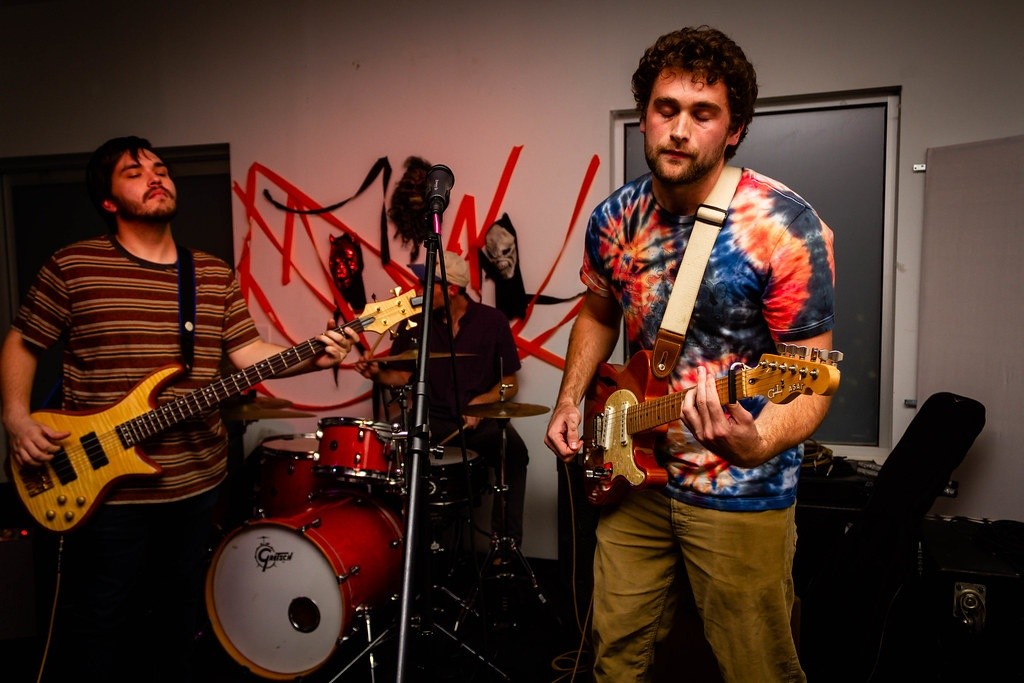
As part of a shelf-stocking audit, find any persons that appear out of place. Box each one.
[0,136,361,683]
[354,252,529,557]
[543,25,836,683]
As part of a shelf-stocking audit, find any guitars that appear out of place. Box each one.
[580,343,845,510]
[6,287,424,535]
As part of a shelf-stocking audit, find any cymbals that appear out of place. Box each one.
[226,395,293,409]
[461,399,552,417]
[225,404,317,420]
[358,346,478,363]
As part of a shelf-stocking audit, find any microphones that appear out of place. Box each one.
[424,164,454,213]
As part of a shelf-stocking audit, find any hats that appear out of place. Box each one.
[406,251,469,288]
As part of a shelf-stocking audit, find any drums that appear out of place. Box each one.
[423,447,481,508]
[254,432,320,510]
[314,416,402,486]
[201,502,408,678]
